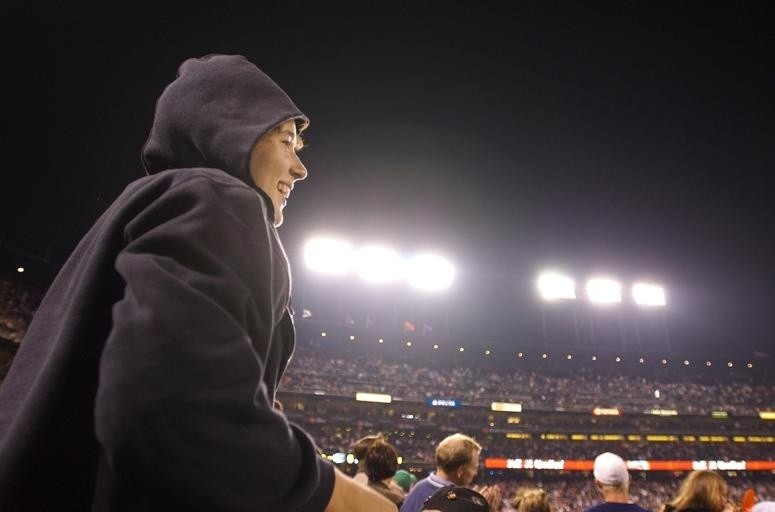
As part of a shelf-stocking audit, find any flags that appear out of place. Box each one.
[403,322,416,332]
[424,324,433,331]
[302,309,311,318]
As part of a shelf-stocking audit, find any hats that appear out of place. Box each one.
[593,451,629,485]
[423,486,489,512]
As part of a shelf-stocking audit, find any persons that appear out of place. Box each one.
[276,351,775,511]
[0,283,51,386]
[1,51,399,512]
